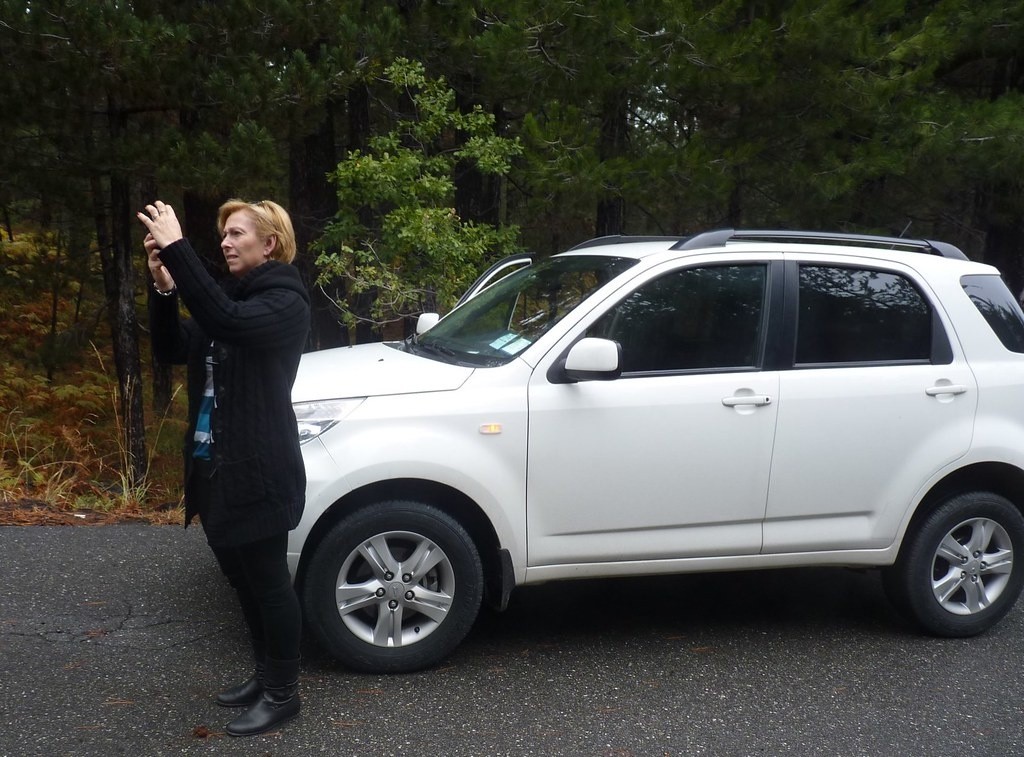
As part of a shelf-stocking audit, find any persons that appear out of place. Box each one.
[137,199,311,736]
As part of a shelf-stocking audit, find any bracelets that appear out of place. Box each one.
[152,280,177,295]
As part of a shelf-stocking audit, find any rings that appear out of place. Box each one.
[151,213,159,220]
[159,210,166,213]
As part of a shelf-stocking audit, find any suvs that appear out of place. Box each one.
[287,226,1024,678]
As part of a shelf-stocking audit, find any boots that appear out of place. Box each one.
[215,670,301,737]
